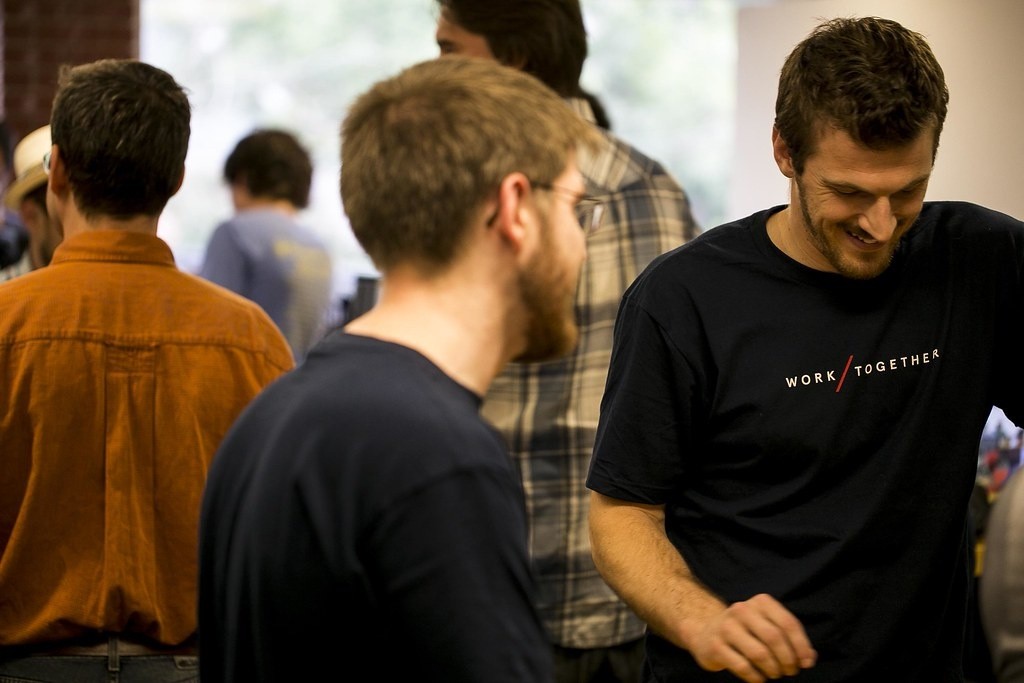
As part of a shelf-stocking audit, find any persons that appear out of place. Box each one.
[195,54,606,682]
[0,56,298,683]
[436,0,707,683]
[0,120,64,283]
[194,127,344,369]
[584,13,1023,683]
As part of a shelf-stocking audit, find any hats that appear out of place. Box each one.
[2,124,52,212]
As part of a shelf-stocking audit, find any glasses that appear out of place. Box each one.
[486,179,606,239]
[43,151,51,173]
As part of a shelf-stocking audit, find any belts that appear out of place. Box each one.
[0,627,199,656]
